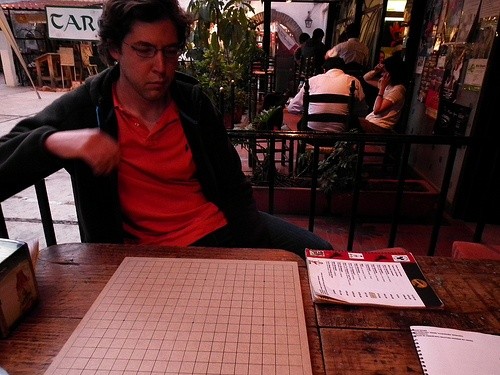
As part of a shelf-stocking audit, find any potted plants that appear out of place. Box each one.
[231,106,440,216]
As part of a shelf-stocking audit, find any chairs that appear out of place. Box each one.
[248,79,410,176]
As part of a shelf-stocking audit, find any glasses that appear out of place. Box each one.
[122,40,182,61]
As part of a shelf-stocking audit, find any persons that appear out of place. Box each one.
[325,26,370,72]
[0,0,332,254]
[288,56,367,147]
[452,59,463,89]
[304,28,325,57]
[359,56,406,134]
[294,33,310,60]
[380,51,385,61]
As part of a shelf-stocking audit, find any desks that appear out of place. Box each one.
[305,249,500,375]
[1,244,325,375]
[33,53,74,90]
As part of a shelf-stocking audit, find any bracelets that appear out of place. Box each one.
[377,94,383,97]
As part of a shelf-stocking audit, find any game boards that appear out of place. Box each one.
[42,257,313,374]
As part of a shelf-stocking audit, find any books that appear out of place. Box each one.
[305,248,444,310]
